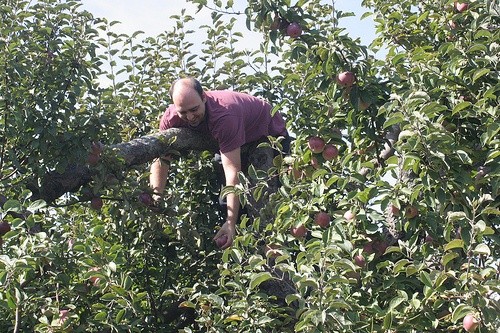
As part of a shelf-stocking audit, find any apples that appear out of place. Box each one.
[0,1,490,333]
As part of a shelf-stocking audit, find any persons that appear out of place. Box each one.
[151,78,291,249]
